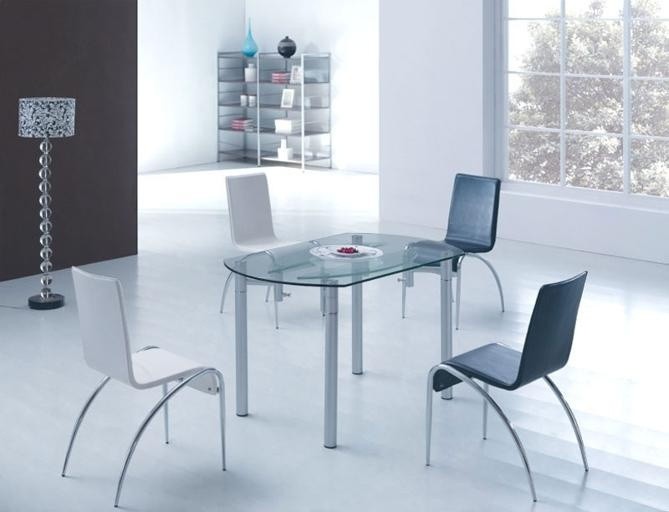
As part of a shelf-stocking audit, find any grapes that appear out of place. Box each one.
[342,247,354,252]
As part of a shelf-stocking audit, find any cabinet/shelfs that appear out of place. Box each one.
[216,51,333,170]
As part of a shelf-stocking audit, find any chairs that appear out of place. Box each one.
[60,265,227,507]
[399,173,505,331]
[424,269,590,503]
[219,172,325,331]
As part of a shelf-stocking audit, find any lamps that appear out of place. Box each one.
[17,97,76,312]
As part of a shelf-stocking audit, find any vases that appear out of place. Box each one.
[278,36,295,58]
[242,18,256,57]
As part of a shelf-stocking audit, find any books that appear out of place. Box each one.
[231,118,254,131]
[271,72,290,84]
[274,118,306,134]
